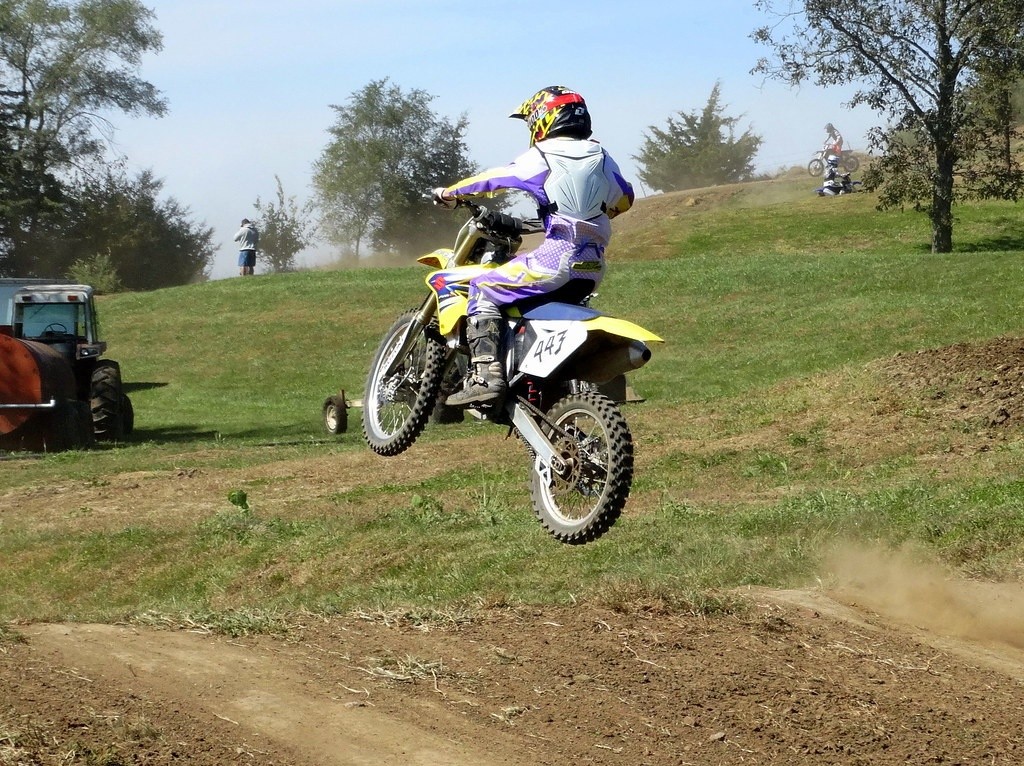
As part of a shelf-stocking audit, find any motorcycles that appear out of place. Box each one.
[808,144,859,177]
[814,172,864,197]
[361,193,666,545]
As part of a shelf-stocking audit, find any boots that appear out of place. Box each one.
[445,316,504,406]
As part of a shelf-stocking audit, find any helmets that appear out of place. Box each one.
[828,155,839,168]
[508,84,593,148]
[824,123,833,133]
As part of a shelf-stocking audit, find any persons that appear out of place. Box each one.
[823,155,849,196]
[234,218,259,275]
[431,85,634,405]
[823,123,843,157]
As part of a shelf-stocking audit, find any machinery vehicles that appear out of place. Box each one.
[0,285,123,443]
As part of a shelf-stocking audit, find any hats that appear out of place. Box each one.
[241,219,250,227]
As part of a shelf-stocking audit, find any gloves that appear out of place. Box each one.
[422,188,457,211]
